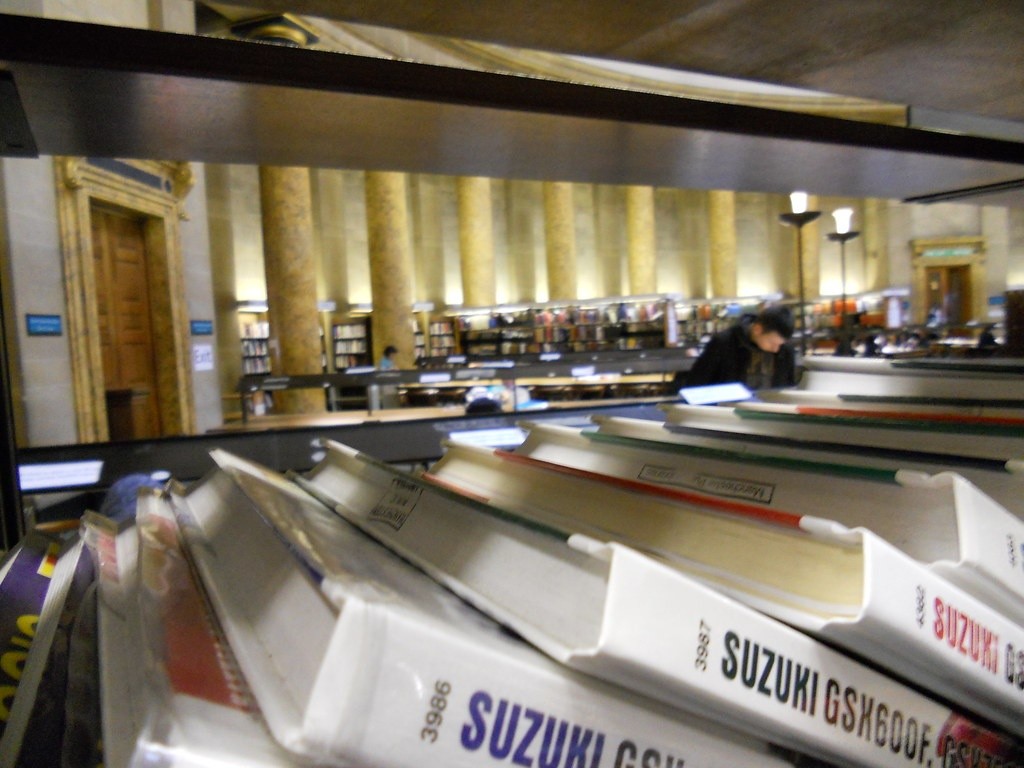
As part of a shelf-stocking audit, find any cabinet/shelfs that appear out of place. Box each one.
[0,0,1024,563]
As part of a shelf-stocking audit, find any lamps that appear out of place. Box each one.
[826,208,860,356]
[777,191,822,356]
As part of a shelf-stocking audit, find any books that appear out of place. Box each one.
[1,357,1024,768]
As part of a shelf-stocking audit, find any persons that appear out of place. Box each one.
[616,303,628,349]
[378,346,403,408]
[686,305,795,389]
